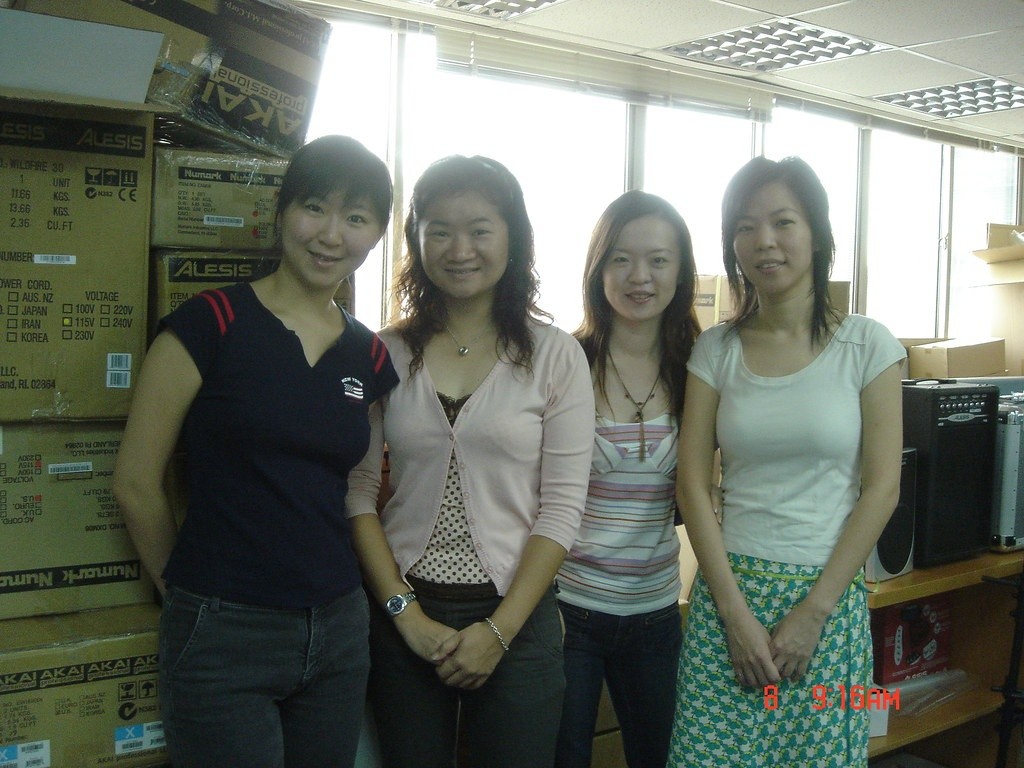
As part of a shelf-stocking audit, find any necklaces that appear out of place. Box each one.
[439,313,492,357]
[605,343,666,463]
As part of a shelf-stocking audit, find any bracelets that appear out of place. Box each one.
[486,617,509,652]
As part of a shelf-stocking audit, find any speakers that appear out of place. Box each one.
[861,383,1001,582]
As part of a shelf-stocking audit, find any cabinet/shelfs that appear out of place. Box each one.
[591,521,1024,768]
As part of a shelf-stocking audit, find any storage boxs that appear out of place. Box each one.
[909,336,1009,379]
[1,0,332,767]
[694,274,852,332]
[972,222,1024,376]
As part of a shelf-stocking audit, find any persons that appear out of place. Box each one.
[109,137,399,768]
[554,188,721,768]
[661,153,908,768]
[343,153,596,768]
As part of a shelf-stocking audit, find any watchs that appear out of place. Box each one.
[382,590,417,619]
